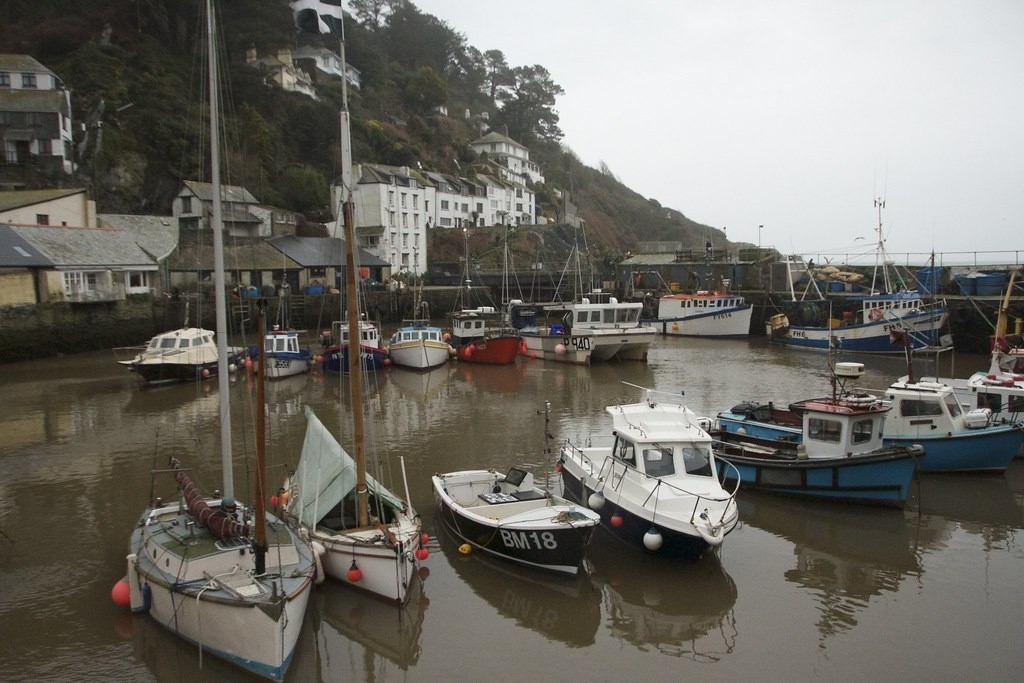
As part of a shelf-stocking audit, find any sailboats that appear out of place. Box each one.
[110,0,429,683]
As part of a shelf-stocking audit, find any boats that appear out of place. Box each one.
[765,162,954,353]
[555,380,741,556]
[638,224,753,339]
[111,163,657,384]
[431,399,601,581]
[696,253,1024,510]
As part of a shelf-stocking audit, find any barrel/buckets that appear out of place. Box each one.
[670,282,681,291]
[915,266,1006,296]
[827,319,841,328]
[551,324,563,335]
[990,335,1006,354]
[539,327,551,335]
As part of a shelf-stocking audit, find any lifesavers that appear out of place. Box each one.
[1002,371,1024,381]
[272,331,287,334]
[1008,348,1024,354]
[847,395,877,408]
[870,309,882,321]
[984,374,1014,385]
